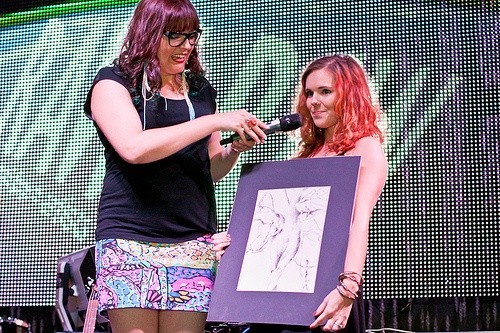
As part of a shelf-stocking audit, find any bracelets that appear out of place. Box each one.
[337,271,365,302]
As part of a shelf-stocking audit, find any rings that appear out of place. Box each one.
[247,116,258,130]
[334,323,343,329]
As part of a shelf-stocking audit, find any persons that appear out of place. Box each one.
[82,1,269,333]
[211,54,390,333]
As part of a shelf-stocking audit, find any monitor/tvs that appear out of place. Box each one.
[56,245,96,313]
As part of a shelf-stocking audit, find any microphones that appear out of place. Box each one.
[220,112,304,145]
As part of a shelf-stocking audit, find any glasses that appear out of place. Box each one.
[162,28,203,47]
